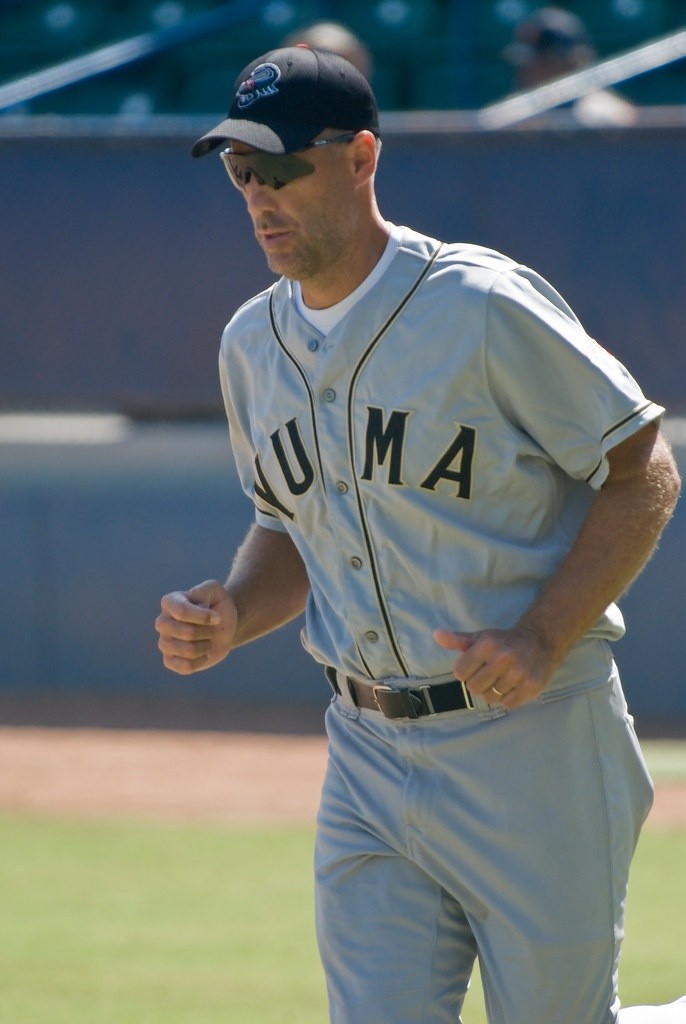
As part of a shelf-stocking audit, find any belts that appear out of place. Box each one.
[333,676,473,719]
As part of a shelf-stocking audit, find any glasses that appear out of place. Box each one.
[220,135,354,190]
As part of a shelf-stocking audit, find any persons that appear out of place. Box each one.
[285,8,636,124]
[155,45,680,1024]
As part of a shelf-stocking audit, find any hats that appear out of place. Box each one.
[190,43,382,158]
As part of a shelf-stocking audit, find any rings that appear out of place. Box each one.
[493,685,503,696]
